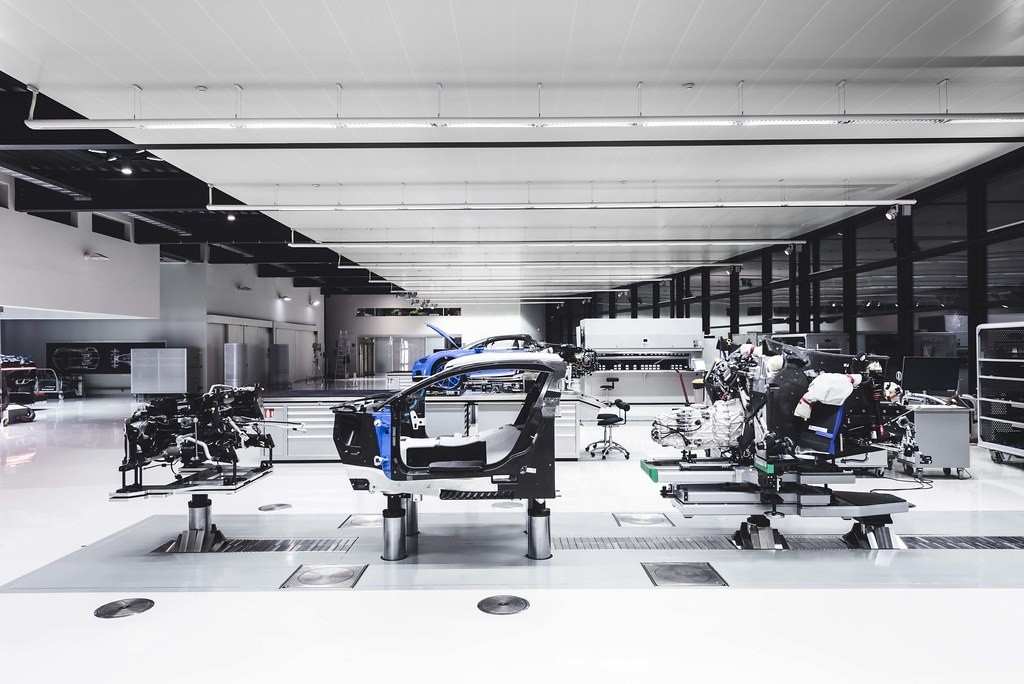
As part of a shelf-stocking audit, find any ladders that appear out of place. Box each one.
[334,329,348,379]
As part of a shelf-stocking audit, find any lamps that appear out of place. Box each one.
[23,78,1024,310]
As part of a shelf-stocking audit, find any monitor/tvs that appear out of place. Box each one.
[901,357,960,391]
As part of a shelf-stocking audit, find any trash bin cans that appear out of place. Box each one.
[691,378,705,404]
